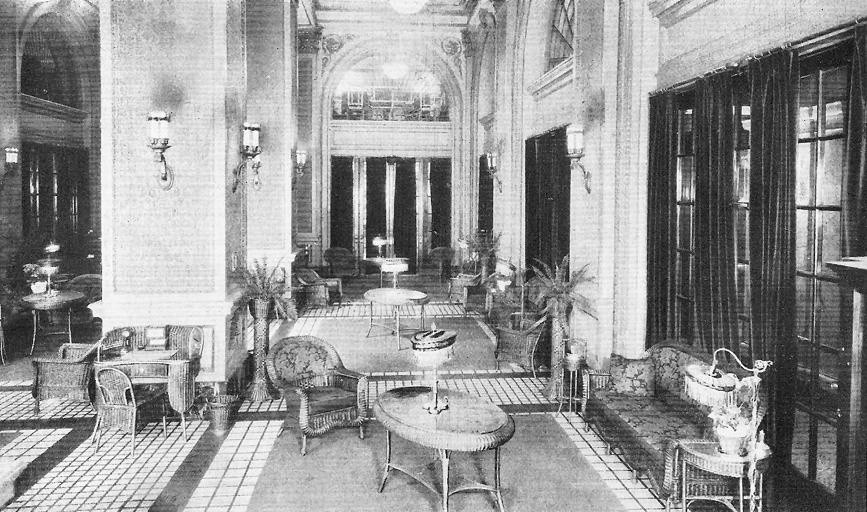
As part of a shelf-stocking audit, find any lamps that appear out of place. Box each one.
[684,346,769,453]
[293,145,307,175]
[232,118,268,190]
[143,108,178,190]
[1,145,23,184]
[564,119,593,191]
[484,145,502,194]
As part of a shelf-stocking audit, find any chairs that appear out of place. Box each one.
[227,228,557,378]
[263,331,368,453]
[0,236,216,463]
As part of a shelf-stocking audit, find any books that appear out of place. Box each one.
[147,339,168,345]
[145,325,168,337]
[144,345,167,351]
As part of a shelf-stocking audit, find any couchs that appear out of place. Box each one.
[577,339,764,512]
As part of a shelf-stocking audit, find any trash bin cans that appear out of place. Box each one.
[207,395,232,436]
[589,368,611,389]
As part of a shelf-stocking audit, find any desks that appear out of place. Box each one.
[663,436,769,512]
[370,384,516,512]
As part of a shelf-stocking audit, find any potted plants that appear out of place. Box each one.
[526,253,599,338]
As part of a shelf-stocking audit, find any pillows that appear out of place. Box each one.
[606,351,656,398]
